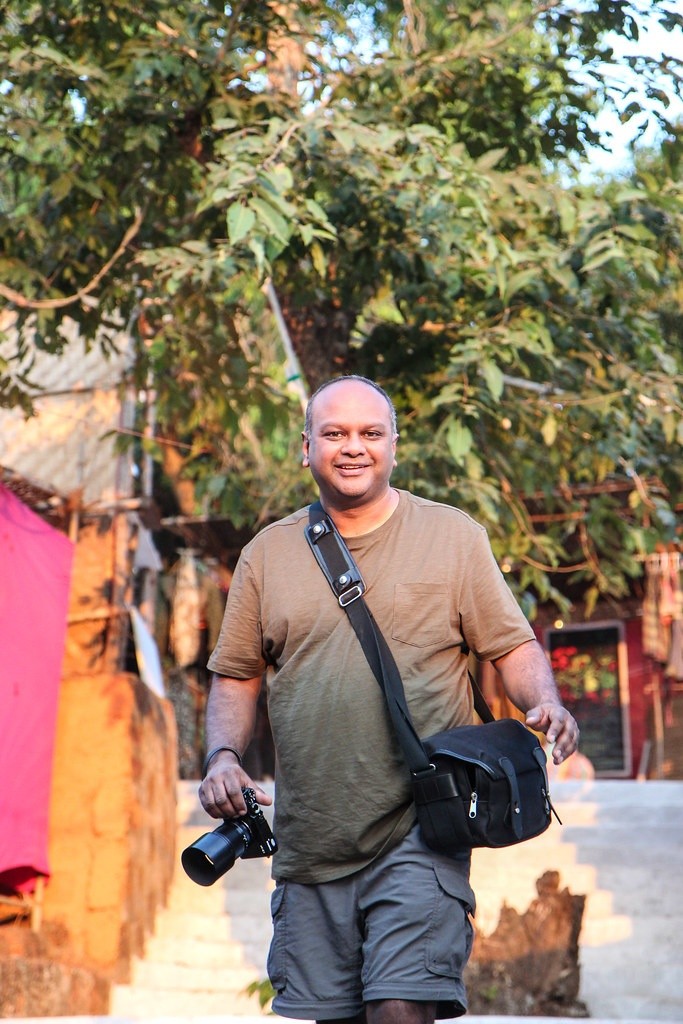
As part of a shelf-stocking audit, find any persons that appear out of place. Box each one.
[199,375,580,1024]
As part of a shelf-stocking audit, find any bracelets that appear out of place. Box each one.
[202,745,243,779]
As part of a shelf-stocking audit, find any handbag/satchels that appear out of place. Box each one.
[410,718,549,853]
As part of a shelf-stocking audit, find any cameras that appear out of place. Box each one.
[181,787,278,887]
